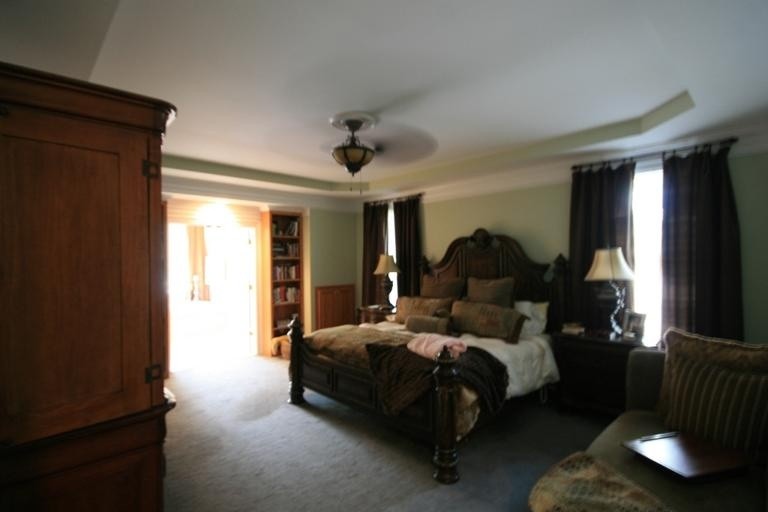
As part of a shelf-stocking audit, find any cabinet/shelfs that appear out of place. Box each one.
[0,58,178,512]
[256,208,304,358]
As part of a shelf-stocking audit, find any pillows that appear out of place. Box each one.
[467,276,515,308]
[395,296,453,324]
[663,352,768,451]
[514,295,548,337]
[658,326,768,451]
[448,299,527,345]
[420,274,465,300]
[405,314,453,336]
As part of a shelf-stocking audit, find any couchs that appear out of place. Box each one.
[584,345,768,512]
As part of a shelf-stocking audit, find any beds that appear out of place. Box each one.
[287,228,573,484]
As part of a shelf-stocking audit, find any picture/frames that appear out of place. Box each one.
[622,311,643,332]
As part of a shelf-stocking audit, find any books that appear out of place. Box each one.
[273,263,300,278]
[273,240,300,257]
[273,219,298,236]
[273,285,299,303]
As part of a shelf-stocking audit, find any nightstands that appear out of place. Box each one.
[355,305,392,326]
[552,312,646,415]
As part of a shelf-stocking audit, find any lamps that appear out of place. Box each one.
[583,246,637,338]
[329,111,375,178]
[372,254,402,310]
